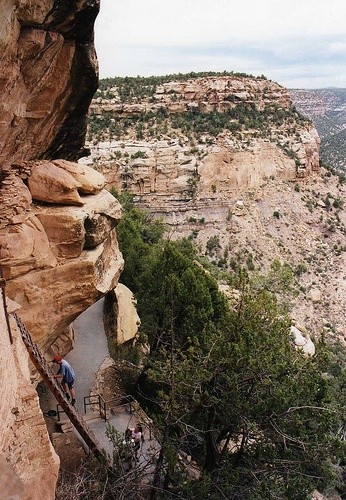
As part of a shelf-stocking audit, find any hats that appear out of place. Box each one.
[52,356,61,363]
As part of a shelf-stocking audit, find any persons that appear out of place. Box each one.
[49,355,76,406]
[132,423,145,462]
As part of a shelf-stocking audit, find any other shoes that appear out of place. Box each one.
[66,393,70,400]
[71,399,75,406]
